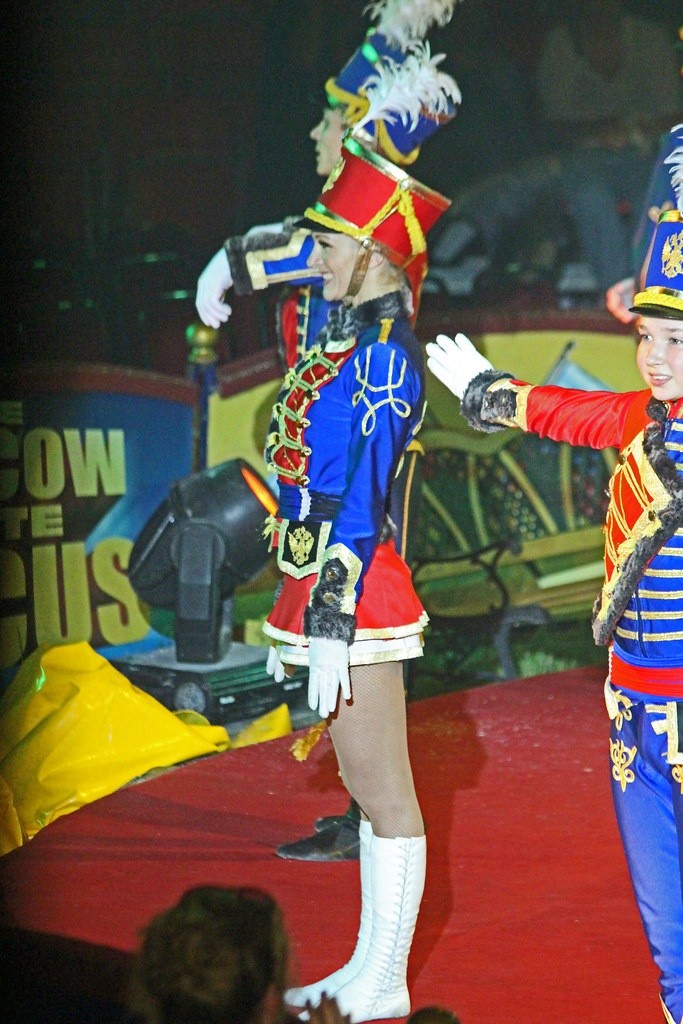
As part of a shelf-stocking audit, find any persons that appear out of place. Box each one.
[129,882,292,1024]
[195,28,464,1024]
[425,125,683,1024]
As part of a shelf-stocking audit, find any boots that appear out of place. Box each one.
[283,820,427,1024]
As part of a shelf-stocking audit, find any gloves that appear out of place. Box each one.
[243,222,283,248]
[424,332,496,401]
[308,637,352,719]
[195,246,234,330]
[267,638,296,684]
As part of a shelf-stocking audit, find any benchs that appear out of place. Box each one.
[404,539,551,701]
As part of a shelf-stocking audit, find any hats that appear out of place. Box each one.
[292,0,461,271]
[628,123,682,321]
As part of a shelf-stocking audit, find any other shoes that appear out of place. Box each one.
[275,811,363,861]
